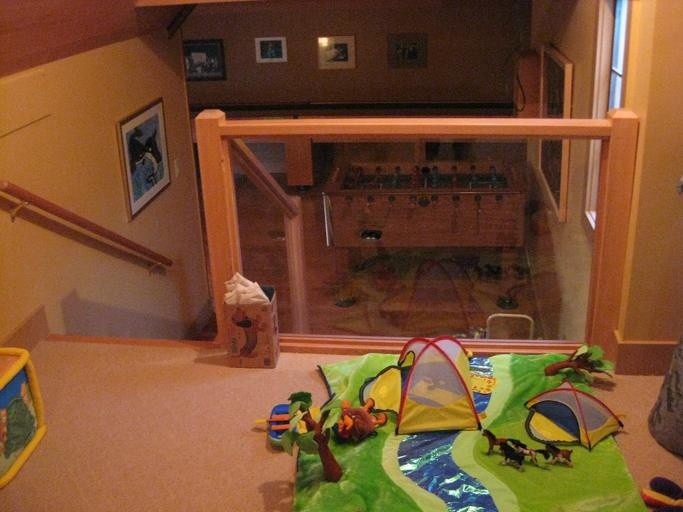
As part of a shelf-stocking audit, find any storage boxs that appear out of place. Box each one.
[223,272,280,368]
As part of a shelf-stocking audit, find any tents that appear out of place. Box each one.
[524,380,624,451]
[396,335,483,435]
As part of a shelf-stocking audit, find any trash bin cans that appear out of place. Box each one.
[359,229,384,258]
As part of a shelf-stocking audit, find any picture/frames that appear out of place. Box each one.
[184,39,228,81]
[535,42,574,222]
[116,96,171,222]
[254,36,288,64]
[312,31,360,74]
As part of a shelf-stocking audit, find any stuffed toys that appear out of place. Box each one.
[254,391,387,482]
